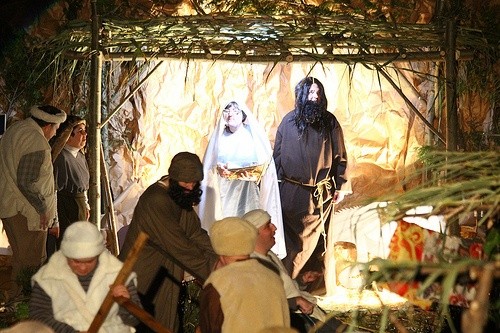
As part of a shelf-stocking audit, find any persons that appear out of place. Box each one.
[273,77,347,305]
[47,116,90,254]
[200,217,291,333]
[118,152,223,333]
[27,221,144,333]
[197,97,287,260]
[0,105,67,283]
[241,209,328,333]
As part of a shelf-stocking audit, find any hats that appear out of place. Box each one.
[242,208,271,228]
[59,221,104,261]
[168,152,204,181]
[208,217,257,256]
